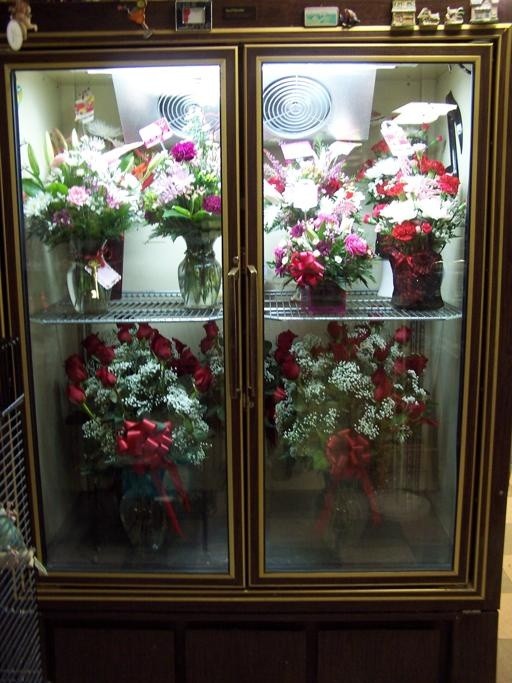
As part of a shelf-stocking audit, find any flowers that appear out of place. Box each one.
[264,321,442,485]
[263,138,376,290]
[63,321,225,482]
[139,117,221,244]
[357,123,467,251]
[19,129,143,251]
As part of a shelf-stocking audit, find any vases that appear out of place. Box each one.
[317,484,371,549]
[300,280,347,316]
[176,229,221,312]
[67,239,120,318]
[386,248,445,311]
[117,465,178,548]
